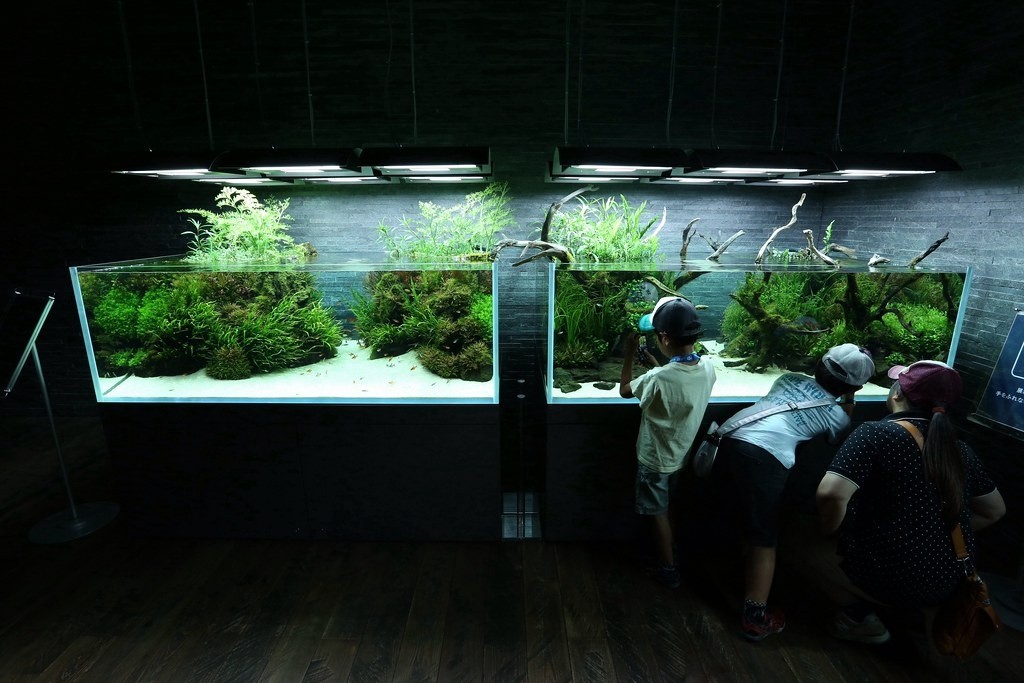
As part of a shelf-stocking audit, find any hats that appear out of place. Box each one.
[888,360,963,411]
[822,343,876,386]
[639,297,701,338]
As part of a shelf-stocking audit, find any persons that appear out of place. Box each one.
[704,343,876,641]
[787,359,1006,644]
[620,302,723,589]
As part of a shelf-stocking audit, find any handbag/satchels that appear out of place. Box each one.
[692,420,723,480]
[932,576,1003,662]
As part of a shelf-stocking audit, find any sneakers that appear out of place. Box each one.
[820,607,890,645]
[742,608,785,642]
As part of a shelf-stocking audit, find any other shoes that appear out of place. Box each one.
[640,560,678,577]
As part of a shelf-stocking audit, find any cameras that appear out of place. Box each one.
[634,334,649,361]
